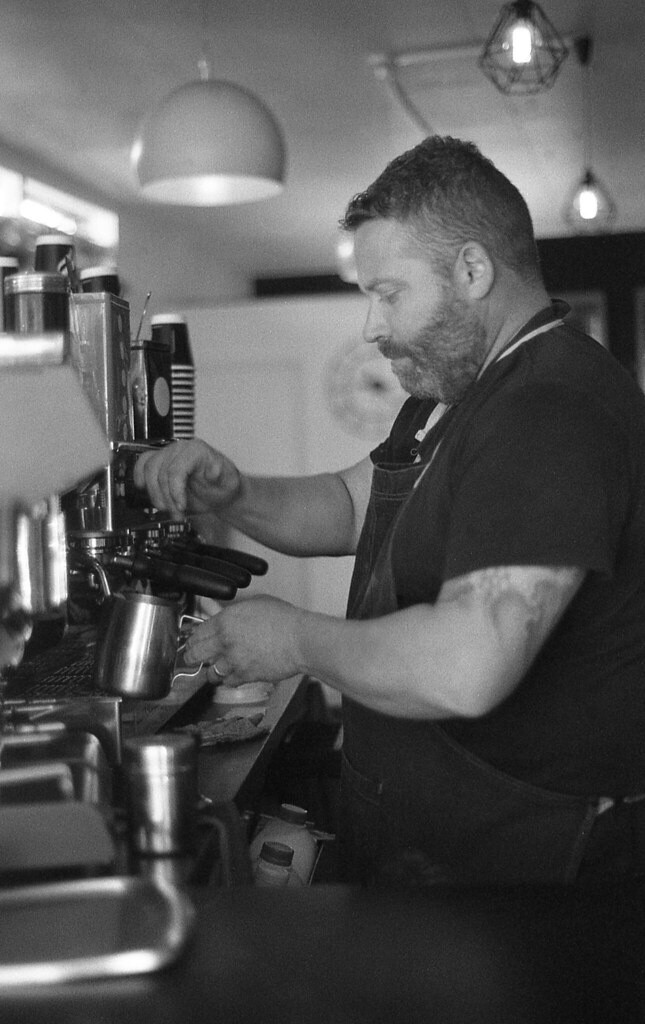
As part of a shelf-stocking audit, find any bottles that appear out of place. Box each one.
[245,839,306,905]
[244,798,320,888]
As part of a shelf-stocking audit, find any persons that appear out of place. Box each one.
[131,136,645,898]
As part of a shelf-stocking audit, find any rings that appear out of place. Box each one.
[214,665,225,678]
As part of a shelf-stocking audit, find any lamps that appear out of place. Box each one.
[130,2,286,212]
[475,1,570,98]
[563,33,618,238]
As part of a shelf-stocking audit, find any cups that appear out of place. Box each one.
[121,731,198,855]
[2,270,70,340]
[33,231,77,290]
[79,262,122,296]
[94,588,213,703]
[211,683,271,706]
[0,255,21,333]
[149,311,198,442]
